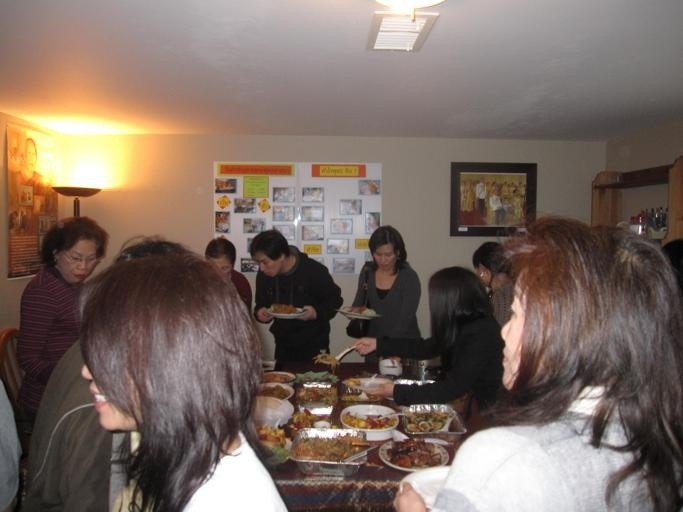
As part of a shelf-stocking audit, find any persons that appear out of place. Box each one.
[355,266,505,408]
[1,378,24,511]
[393,216,682,511]
[19,216,106,437]
[12,137,48,213]
[460,173,523,225]
[348,224,421,367]
[202,235,252,332]
[79,247,285,512]
[473,240,516,327]
[26,237,187,511]
[248,230,342,373]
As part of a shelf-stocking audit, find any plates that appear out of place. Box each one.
[347,377,391,392]
[377,440,449,473]
[338,403,399,441]
[256,381,293,400]
[263,370,296,385]
[263,306,306,319]
[334,307,380,321]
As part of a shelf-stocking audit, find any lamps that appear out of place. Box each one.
[51,186,101,219]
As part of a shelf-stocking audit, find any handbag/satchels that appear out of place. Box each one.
[345,302,371,339]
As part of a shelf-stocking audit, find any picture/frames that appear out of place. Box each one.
[450,162,537,237]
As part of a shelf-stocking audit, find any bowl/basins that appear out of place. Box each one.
[375,358,405,377]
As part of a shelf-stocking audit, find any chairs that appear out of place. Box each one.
[0,328,27,408]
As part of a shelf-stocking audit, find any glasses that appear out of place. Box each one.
[61,252,102,266]
